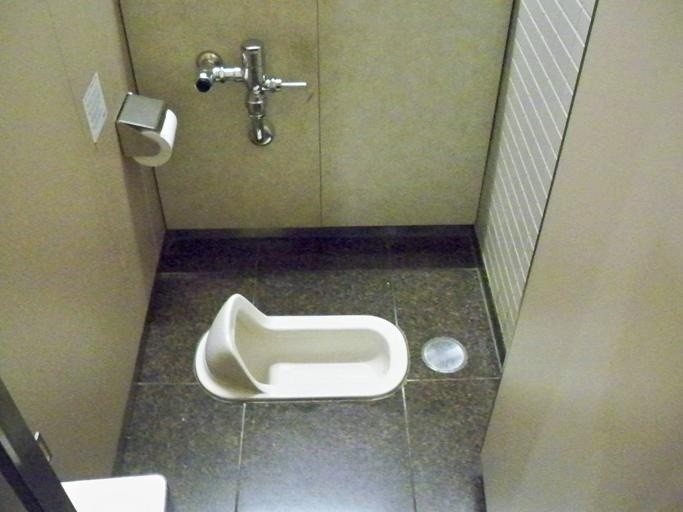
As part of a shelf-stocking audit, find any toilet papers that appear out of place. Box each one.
[131,107,180,169]
